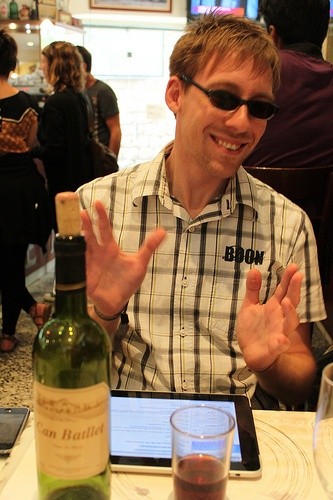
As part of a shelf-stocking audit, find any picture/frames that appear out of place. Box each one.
[90,0,172,13]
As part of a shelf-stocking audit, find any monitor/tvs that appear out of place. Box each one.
[186,0,261,28]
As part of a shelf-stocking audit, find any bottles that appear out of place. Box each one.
[10,0,18,19]
[31,191,111,500]
[32,0,39,20]
[0,0,10,19]
[19,5,31,20]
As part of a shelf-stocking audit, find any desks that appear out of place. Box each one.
[0,410,333,500]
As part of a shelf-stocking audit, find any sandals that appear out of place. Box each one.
[32,303,52,335]
[0,334,19,353]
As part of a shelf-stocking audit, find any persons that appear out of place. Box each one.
[75,46,121,158]
[242,0,333,342]
[48,6,327,408]
[0,28,52,353]
[36,41,119,235]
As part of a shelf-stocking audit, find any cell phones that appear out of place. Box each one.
[0,407,29,457]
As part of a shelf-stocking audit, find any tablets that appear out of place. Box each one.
[108,389,262,479]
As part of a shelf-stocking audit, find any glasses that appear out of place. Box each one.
[178,73,281,122]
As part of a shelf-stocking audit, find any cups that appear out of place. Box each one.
[313,362,333,500]
[169,403,236,500]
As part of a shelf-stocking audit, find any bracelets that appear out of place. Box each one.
[93,301,128,321]
